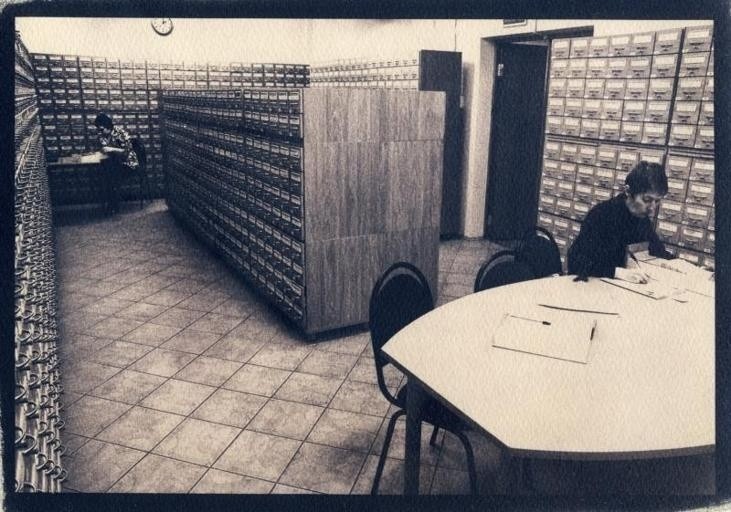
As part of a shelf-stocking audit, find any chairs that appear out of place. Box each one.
[474,224,584,293]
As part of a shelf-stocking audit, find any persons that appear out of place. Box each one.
[95,112,140,213]
[568,162,678,285]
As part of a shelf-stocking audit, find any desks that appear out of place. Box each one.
[382,268,720,498]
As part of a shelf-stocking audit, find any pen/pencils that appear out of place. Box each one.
[629,252,646,283]
[511,314,551,325]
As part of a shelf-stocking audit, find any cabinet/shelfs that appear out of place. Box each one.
[33,61,309,213]
[308,50,460,242]
[158,85,445,339]
[7,37,68,494]
[529,21,728,275]
